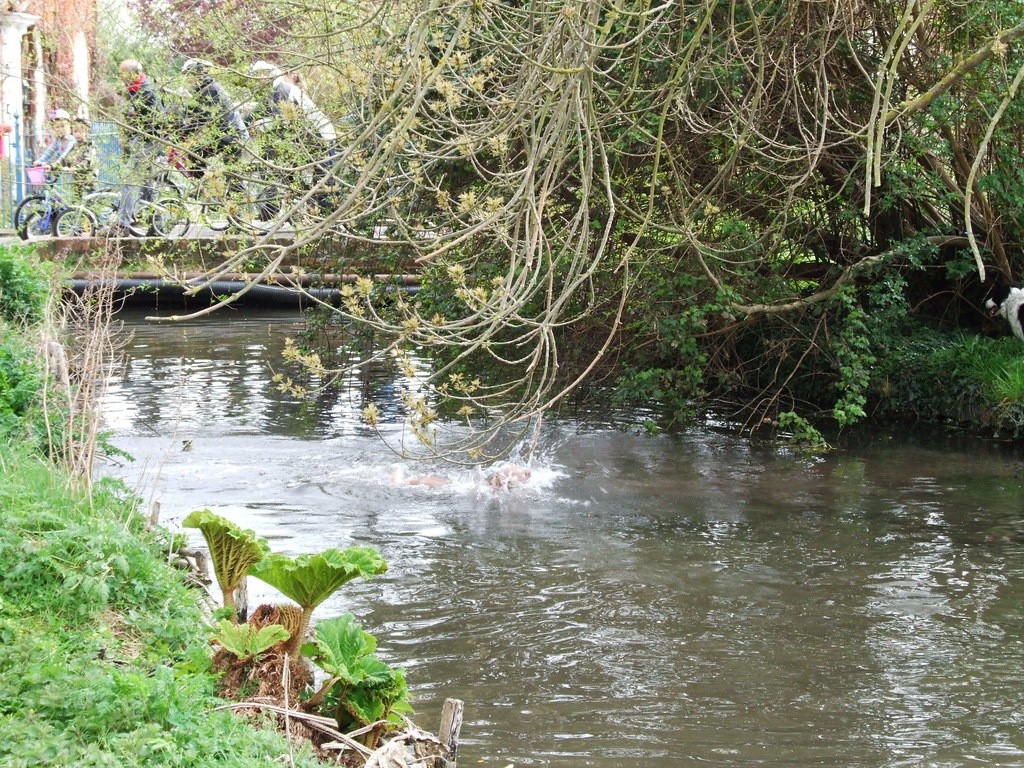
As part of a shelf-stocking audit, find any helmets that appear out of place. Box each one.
[50,109,71,122]
[182,58,213,75]
[250,61,278,78]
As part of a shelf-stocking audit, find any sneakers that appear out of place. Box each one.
[102,224,129,236]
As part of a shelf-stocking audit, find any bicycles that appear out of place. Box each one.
[15,129,375,241]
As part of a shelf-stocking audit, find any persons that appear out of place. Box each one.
[32,58,337,237]
